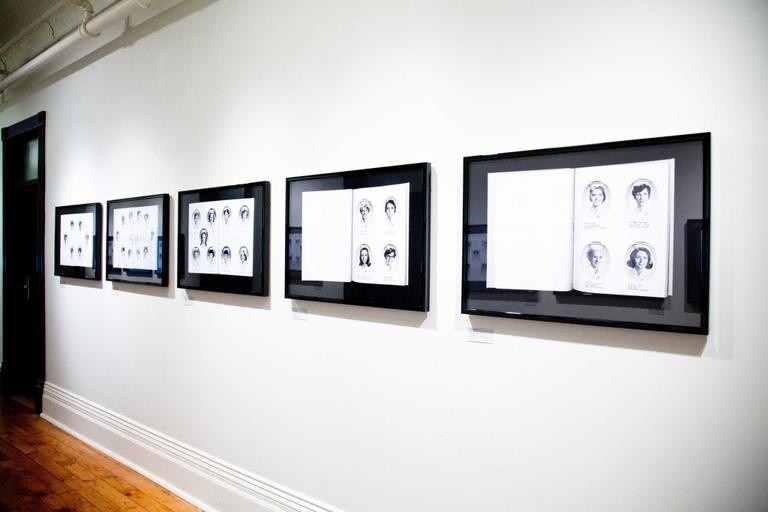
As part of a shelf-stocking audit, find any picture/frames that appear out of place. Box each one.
[54,200,104,282]
[283,161,433,315]
[104,192,170,289]
[459,126,713,334]
[174,180,272,298]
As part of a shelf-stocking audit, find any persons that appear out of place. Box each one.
[585,180,612,211]
[358,197,372,224]
[624,240,655,277]
[185,203,252,266]
[381,197,398,224]
[60,218,90,262]
[356,243,372,272]
[627,176,655,211]
[382,243,397,271]
[112,209,156,262]
[584,238,610,276]
